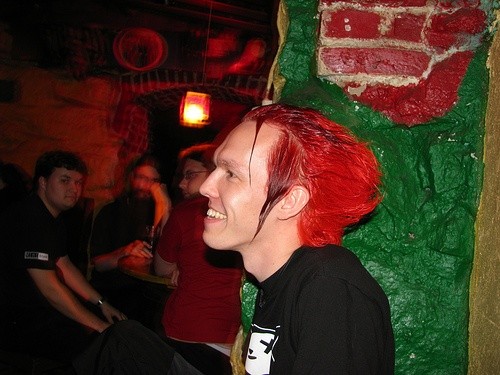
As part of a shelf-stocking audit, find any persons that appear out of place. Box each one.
[154,143,245,375]
[61,103,395,375]
[0,160,31,211]
[0,149,128,360]
[83,147,171,329]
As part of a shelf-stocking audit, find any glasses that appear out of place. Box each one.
[185,170,210,179]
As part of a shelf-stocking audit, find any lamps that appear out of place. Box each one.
[179,91,212,129]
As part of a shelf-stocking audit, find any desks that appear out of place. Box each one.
[118,256,173,285]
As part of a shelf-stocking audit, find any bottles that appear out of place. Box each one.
[146,226,156,254]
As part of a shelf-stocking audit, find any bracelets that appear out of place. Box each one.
[95,297,107,308]
